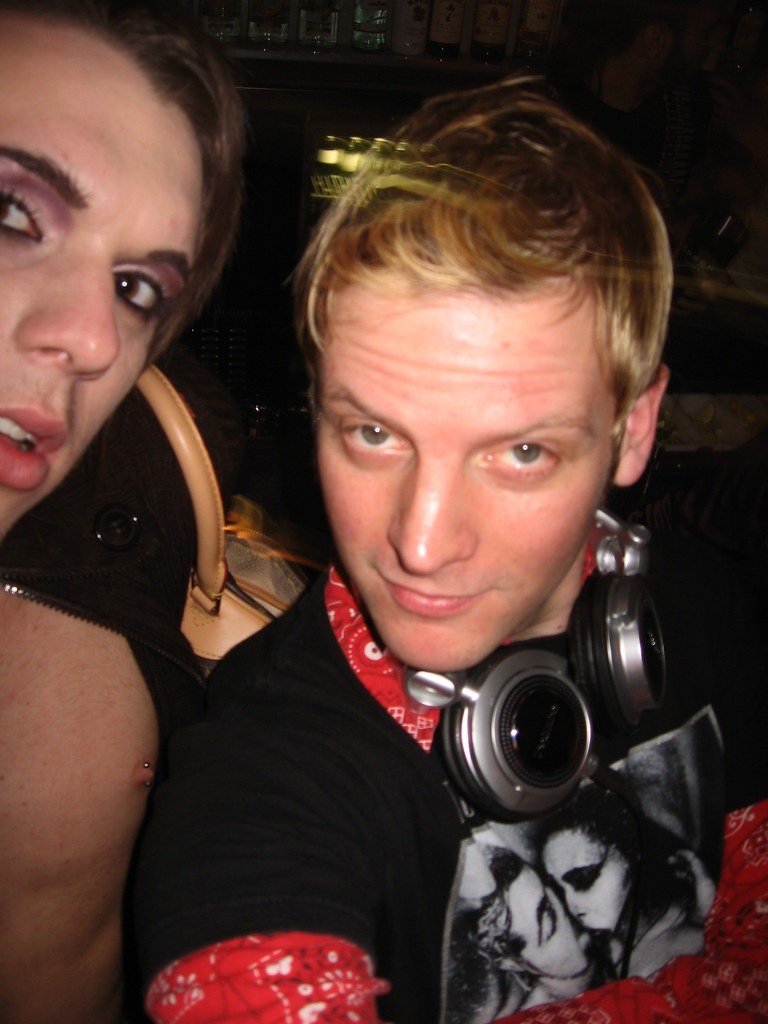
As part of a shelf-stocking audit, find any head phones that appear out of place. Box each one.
[405,506,670,824]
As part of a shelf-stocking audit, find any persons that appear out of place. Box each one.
[535,782,720,980]
[122,76,768,1024]
[0,0,250,1024]
[449,855,614,1021]
